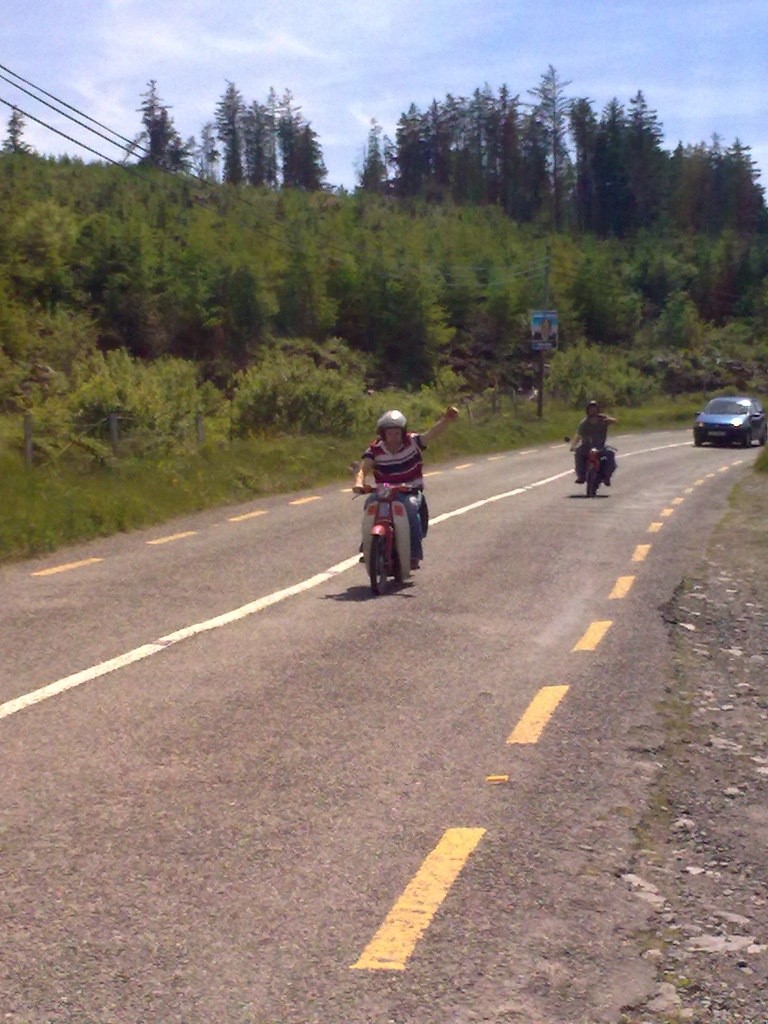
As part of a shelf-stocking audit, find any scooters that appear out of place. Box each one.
[349,477,423,598]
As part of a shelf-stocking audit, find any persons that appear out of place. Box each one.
[352,404,460,570]
[570,400,618,486]
[734,404,744,413]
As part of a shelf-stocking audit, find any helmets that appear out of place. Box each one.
[586,400,601,415]
[377,410,408,432]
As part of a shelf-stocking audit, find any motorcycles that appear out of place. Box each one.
[564,436,617,495]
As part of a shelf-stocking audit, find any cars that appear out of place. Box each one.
[693,396,765,448]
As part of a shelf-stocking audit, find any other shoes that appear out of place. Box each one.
[411,560,417,570]
[576,477,585,483]
[604,478,611,486]
[359,557,365,562]
